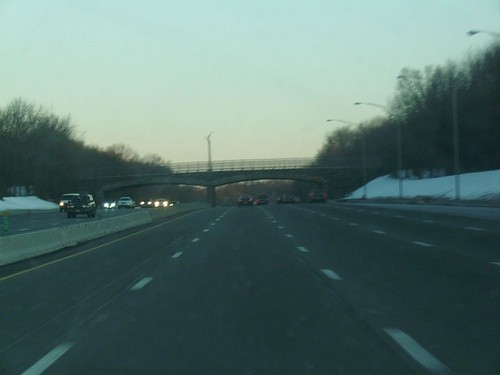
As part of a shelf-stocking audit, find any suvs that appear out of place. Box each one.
[60,193,96,217]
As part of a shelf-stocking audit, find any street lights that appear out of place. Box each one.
[354,102,403,201]
[397,75,461,202]
[326,119,367,200]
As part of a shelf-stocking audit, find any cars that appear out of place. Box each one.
[237,190,328,206]
[104,196,179,210]
